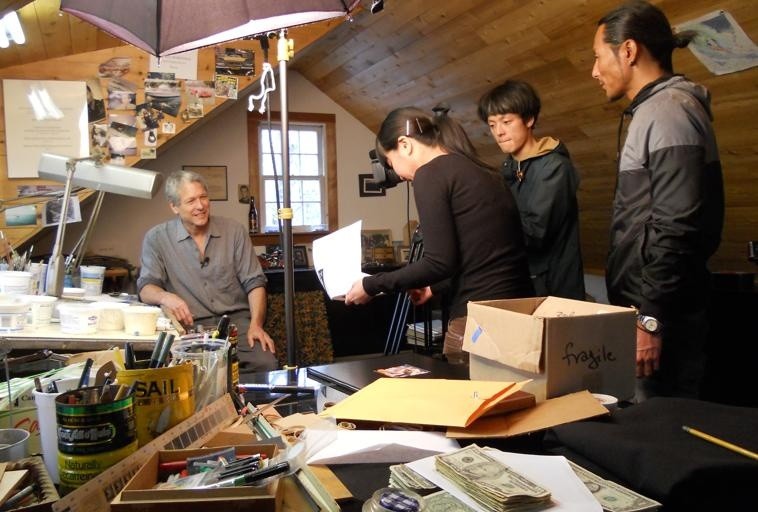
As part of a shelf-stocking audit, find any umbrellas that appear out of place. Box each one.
[57,1,357,364]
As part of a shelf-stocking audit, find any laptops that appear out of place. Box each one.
[306,350,469,392]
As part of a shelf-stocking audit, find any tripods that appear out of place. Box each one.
[381,223,449,354]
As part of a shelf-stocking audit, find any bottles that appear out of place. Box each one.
[247,195,259,236]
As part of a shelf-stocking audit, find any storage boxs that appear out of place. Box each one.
[461,295,638,406]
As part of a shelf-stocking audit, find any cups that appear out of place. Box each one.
[170,339,232,414]
[31,378,119,487]
[0,294,161,336]
[0,271,32,292]
[80,265,106,294]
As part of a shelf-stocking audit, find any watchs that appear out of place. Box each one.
[636,314,660,332]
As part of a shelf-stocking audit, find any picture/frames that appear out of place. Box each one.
[293,245,309,268]
[358,174,386,197]
[181,165,227,201]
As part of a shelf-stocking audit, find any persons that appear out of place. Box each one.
[464,461,497,482]
[238,185,252,204]
[338,101,530,352]
[133,172,278,373]
[83,84,180,159]
[475,81,592,300]
[585,480,602,494]
[587,3,729,406]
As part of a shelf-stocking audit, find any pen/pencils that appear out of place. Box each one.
[236,384,315,393]
[159,454,290,488]
[0,483,39,512]
[58,376,139,404]
[680,425,758,461]
[125,315,234,414]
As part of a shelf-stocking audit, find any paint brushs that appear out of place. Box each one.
[34,356,94,393]
[0,231,34,271]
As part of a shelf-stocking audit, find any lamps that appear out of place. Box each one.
[37,146,166,297]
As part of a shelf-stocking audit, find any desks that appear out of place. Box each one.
[104,266,128,276]
[237,351,758,512]
